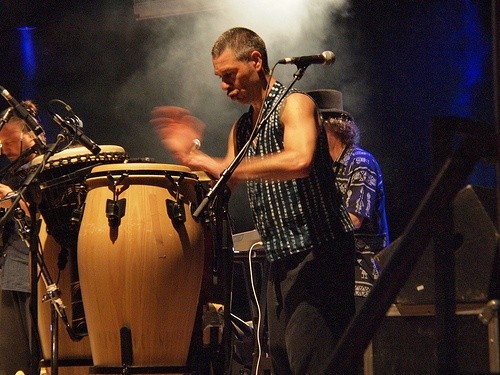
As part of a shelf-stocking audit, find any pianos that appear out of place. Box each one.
[233,229,265,375]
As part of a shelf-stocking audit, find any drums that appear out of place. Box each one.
[29,214,93,375]
[32,145,126,336]
[77,162,204,375]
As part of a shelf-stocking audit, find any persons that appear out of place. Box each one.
[151,26,365,375]
[302,90,390,318]
[0,100,46,375]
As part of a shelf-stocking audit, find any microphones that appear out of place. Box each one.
[0,85,44,136]
[49,108,102,154]
[278,50,336,65]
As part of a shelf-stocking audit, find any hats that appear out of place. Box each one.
[306,90,353,122]
